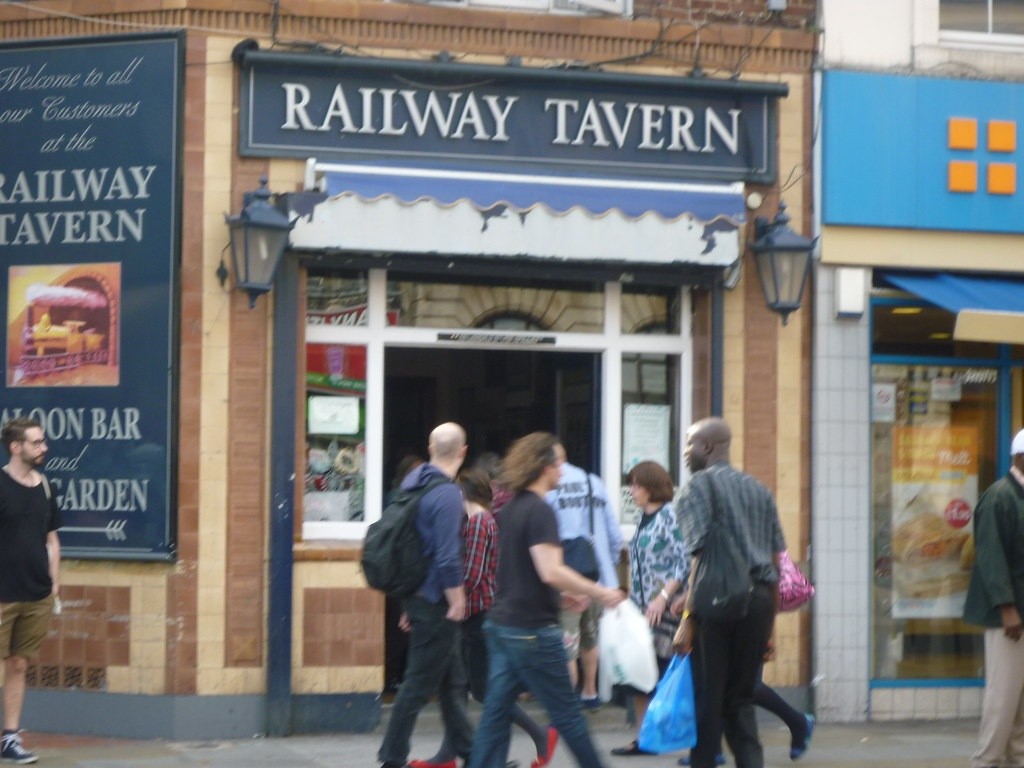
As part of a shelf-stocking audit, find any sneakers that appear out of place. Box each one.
[0,729,39,764]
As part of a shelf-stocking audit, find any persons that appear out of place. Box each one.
[0,418,61,764]
[969,429,1024,768]
[377,417,815,768]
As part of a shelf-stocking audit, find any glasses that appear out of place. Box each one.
[20,436,48,447]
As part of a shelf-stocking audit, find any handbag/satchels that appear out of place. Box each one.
[599,597,659,700]
[780,550,815,612]
[685,470,749,623]
[638,654,697,751]
[562,536,601,583]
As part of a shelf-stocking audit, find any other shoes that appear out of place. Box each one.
[678,754,727,766]
[611,740,658,755]
[407,759,457,768]
[790,712,816,761]
[530,726,558,768]
[580,696,601,711]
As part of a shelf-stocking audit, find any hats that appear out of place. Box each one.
[1009,427,1024,455]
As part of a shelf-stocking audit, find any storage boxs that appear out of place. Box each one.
[303,477,364,523]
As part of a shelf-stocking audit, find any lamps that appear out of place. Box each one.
[216,173,304,311]
[747,200,822,326]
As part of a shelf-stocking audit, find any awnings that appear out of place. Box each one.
[879,272,1024,344]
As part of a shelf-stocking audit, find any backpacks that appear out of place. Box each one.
[358,478,454,602]
